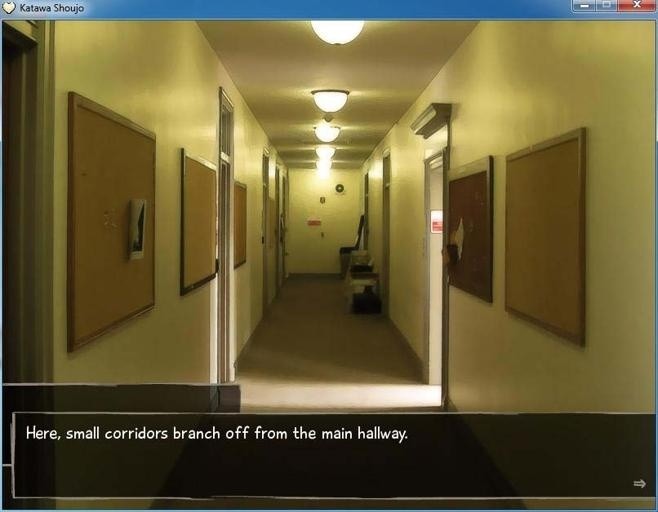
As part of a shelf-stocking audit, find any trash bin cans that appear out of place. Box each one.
[340,247,381,315]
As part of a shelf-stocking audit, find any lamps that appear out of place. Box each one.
[310,89,349,143]
